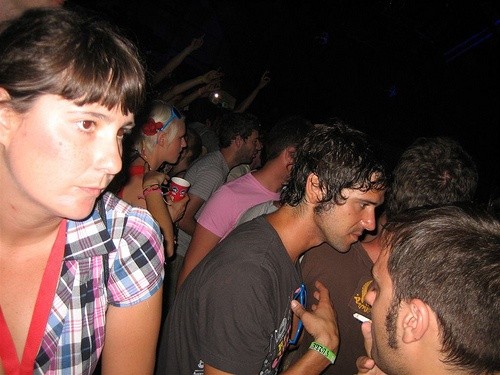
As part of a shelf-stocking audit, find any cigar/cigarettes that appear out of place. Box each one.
[352,312,372,325]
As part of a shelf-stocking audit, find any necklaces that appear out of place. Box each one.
[136,152,156,173]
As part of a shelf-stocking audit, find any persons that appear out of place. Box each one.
[0,0,500,375]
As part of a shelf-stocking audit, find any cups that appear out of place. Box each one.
[168,177,191,203]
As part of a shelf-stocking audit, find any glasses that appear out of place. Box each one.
[155,103,182,144]
[289,282,307,344]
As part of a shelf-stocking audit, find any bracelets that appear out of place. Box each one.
[308,340,337,365]
[143,183,163,196]
